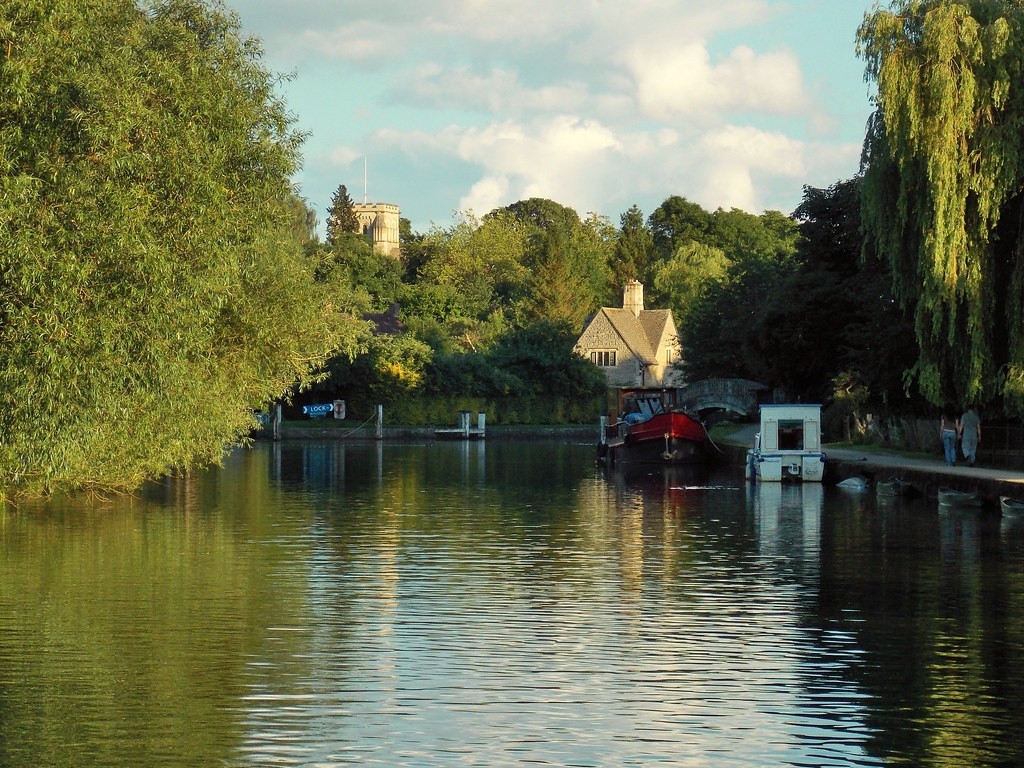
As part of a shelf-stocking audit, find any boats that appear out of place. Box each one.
[597,399,716,465]
[745,403,826,481]
[437,426,486,441]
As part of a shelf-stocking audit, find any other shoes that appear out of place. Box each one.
[952,462,957,467]
[969,464,974,467]
[964,456,970,461]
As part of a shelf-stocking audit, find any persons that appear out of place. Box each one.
[957,403,982,467]
[939,402,959,467]
